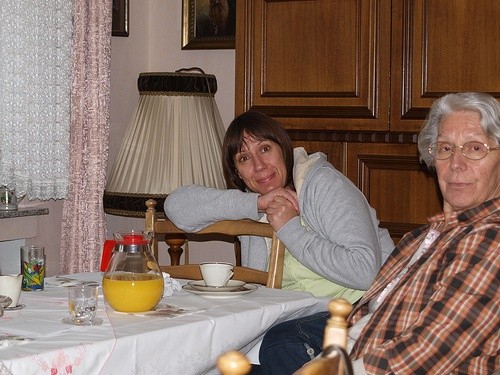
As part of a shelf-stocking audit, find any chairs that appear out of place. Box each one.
[144,199,286,288]
[220,297,353,374]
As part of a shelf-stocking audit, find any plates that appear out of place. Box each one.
[182,284,258,298]
[188,280,247,292]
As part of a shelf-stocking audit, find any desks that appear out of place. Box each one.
[0,272,333,375]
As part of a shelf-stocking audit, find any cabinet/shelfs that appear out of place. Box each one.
[236,0,500,260]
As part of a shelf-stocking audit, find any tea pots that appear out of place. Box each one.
[0,185,26,210]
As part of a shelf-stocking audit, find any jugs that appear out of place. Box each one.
[100,229,164,312]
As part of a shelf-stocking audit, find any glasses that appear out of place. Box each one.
[428,142,489,160]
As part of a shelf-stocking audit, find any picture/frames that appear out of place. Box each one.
[111,0,130,36]
[181,0,236,50]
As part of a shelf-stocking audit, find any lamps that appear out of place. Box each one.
[104,68,227,254]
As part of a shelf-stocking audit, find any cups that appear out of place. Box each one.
[0,273,23,307]
[20,245,46,292]
[67,285,99,325]
[199,261,235,286]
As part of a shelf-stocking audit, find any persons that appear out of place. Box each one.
[163,109,396,311]
[258,93,500,375]
